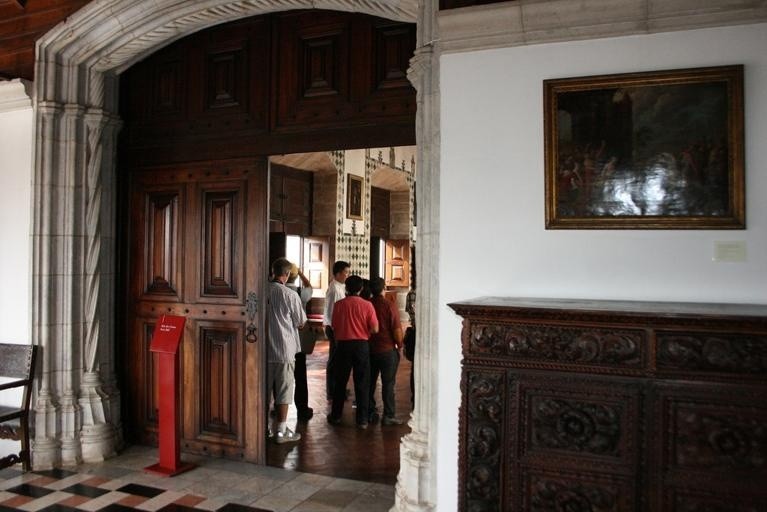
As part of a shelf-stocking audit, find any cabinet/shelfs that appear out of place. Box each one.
[270,161,314,238]
[442,290,764,512]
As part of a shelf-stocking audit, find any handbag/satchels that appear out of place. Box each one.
[299,328,315,354]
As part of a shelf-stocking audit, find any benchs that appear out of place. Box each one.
[397,293,413,323]
[304,296,326,336]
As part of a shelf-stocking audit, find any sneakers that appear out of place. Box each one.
[267,427,301,443]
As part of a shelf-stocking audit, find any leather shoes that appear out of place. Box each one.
[382,415,402,425]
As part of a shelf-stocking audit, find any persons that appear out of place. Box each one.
[558,139,727,218]
[266,258,416,444]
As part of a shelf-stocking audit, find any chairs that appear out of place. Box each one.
[0,340,40,479]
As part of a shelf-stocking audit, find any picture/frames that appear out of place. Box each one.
[345,170,365,220]
[539,63,744,234]
